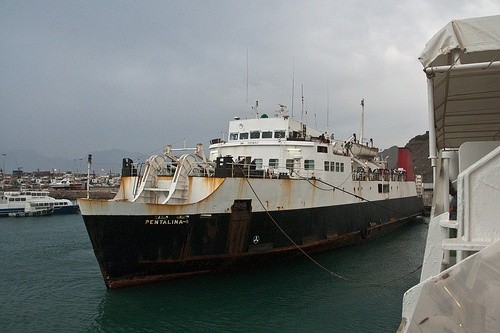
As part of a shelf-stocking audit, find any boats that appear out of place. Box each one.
[0,167,121,189]
[0,190,80,218]
[80,52,425,292]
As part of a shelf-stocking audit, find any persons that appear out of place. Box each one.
[251,158,257,170]
[238,158,245,168]
[345,141,350,155]
[449,194,458,238]
[318,131,335,144]
